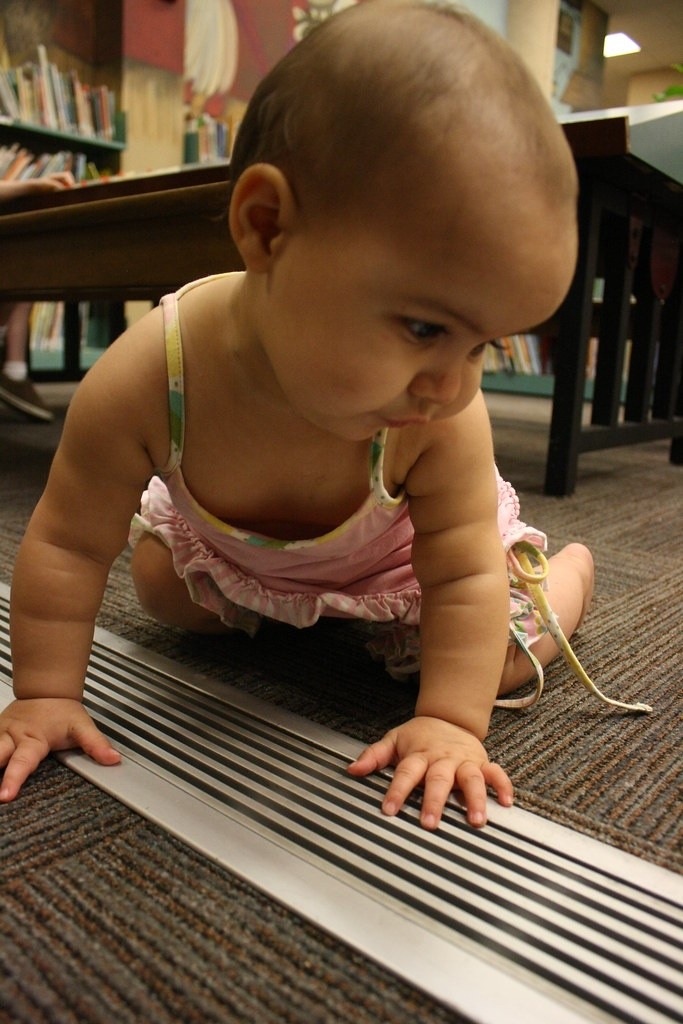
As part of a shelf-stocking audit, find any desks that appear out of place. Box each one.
[0,106,681,498]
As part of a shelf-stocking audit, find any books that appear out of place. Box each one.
[480,334,663,380]
[1,44,231,351]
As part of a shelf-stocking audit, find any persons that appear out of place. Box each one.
[0,0,594,832]
[1,171,75,425]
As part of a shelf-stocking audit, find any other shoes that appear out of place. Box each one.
[0,373,53,422]
